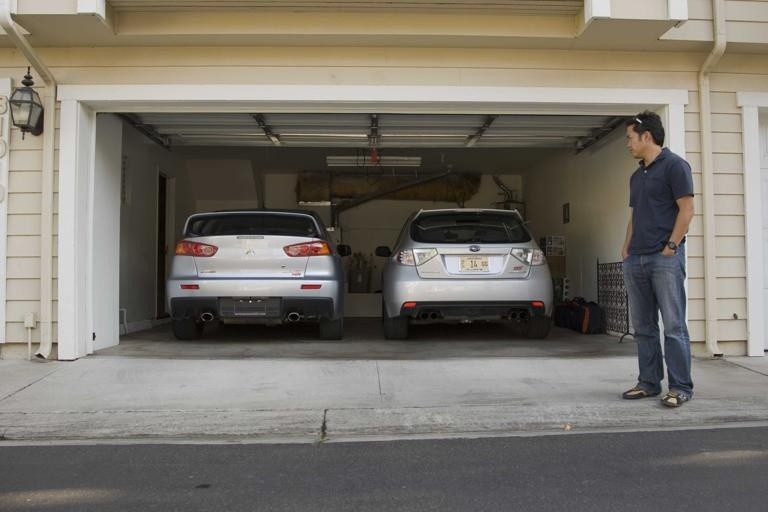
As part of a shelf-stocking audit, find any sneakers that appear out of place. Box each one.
[662,394,693,407]
[623,386,661,398]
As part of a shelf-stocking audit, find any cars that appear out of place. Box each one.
[166,209,350,341]
[375,209,554,341]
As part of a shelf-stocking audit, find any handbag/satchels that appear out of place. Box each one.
[563,295,604,334]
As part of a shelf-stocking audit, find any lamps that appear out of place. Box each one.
[327,156,421,167]
[8,66,44,141]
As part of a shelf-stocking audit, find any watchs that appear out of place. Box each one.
[665,241,678,254]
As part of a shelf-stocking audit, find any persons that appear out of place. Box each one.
[619,108,696,409]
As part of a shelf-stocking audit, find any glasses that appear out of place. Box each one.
[629,113,641,124]
